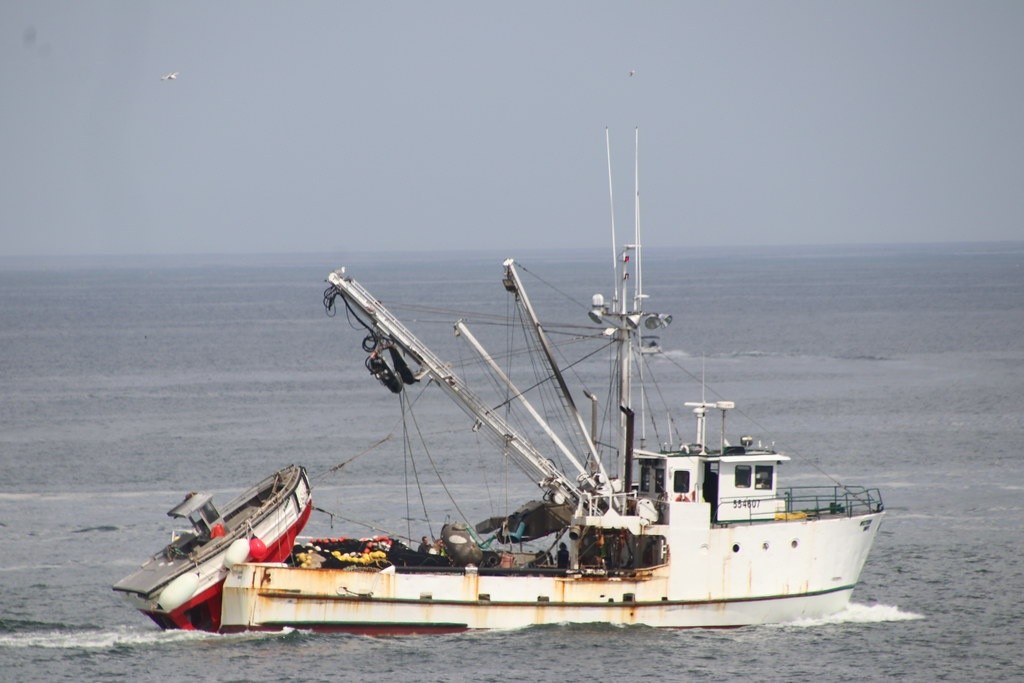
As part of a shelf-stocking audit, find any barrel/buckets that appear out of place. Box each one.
[224,538,250,568]
[159,571,200,610]
[250,536,267,558]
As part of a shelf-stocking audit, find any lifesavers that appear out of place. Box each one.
[676,495,690,502]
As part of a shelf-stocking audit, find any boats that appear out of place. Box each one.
[113,464,316,632]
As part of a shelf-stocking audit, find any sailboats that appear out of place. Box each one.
[216,123,886,635]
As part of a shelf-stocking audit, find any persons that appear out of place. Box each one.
[555,542,569,569]
[418,536,443,554]
